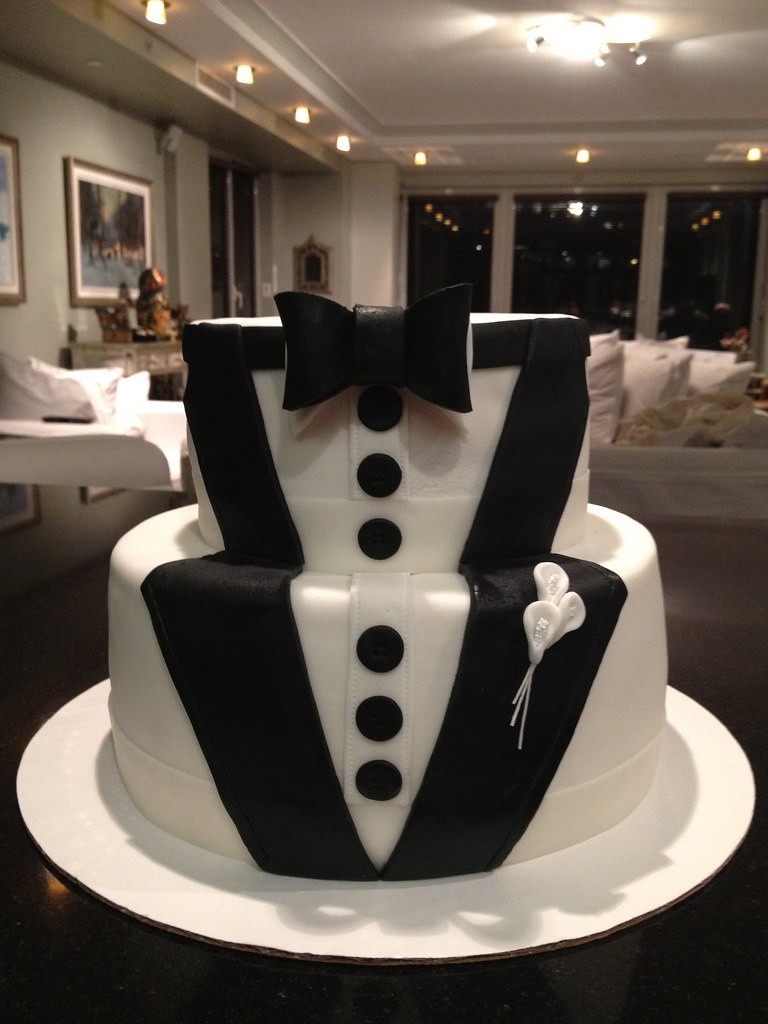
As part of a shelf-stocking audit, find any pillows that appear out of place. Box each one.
[585,331,755,446]
[0,351,150,436]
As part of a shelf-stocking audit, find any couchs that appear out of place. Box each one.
[0,400,189,491]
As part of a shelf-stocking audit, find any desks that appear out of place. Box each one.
[0,483,768,1024]
[68,341,188,398]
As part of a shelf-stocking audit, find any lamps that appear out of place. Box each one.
[413,151,427,166]
[595,52,611,67]
[235,66,254,84]
[294,107,310,124]
[575,150,589,164]
[630,40,646,67]
[336,136,351,152]
[746,148,761,161]
[144,0,168,24]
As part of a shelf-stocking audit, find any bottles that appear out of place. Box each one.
[117,281,138,342]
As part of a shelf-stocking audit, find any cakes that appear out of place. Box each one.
[104,284,670,881]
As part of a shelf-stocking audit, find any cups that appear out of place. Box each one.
[98,307,126,344]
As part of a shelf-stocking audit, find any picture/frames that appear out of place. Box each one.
[0,483,42,538]
[64,154,156,308]
[0,132,28,304]
[80,487,128,504]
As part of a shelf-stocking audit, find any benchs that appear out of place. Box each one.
[587,409,767,520]
